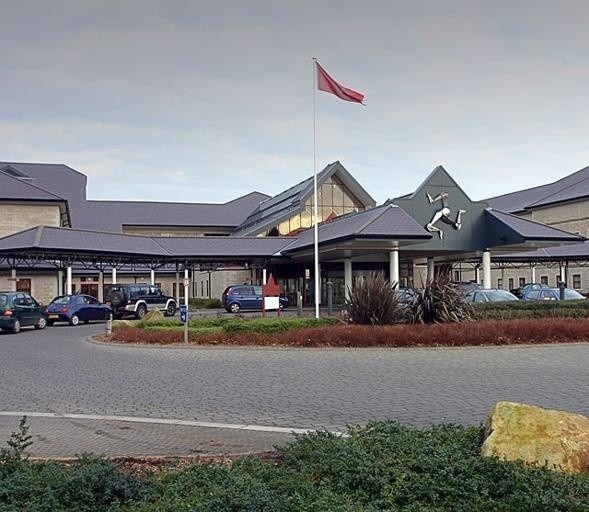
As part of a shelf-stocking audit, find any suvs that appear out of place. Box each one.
[222,284,289,314]
[109,281,178,320]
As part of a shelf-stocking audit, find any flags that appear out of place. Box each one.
[315,62,364,105]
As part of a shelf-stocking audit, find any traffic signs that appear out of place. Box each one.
[179,304,187,324]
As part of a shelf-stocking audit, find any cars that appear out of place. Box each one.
[0,290,48,333]
[392,281,587,313]
[44,294,114,326]
